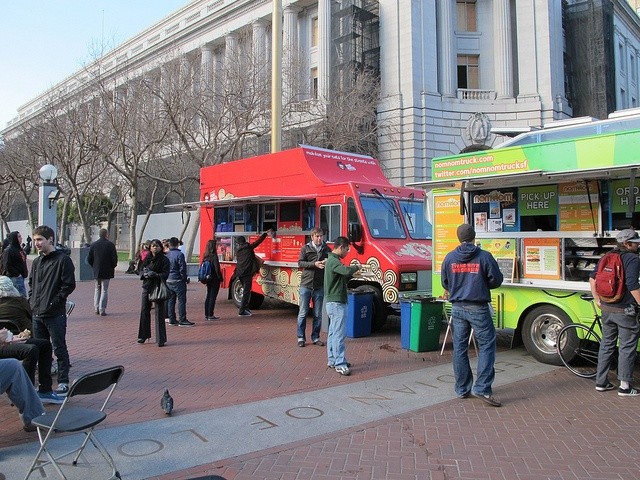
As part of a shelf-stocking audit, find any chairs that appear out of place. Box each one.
[25,365,125,480]
[372,219,386,232]
[65,302,75,318]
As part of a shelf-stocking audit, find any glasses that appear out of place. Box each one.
[626,232,638,240]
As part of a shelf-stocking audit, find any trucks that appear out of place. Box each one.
[432,107,640,366]
[200,144,432,309]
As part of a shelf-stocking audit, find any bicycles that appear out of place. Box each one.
[556,293,618,379]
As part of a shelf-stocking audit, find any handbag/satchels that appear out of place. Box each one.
[148,274,171,302]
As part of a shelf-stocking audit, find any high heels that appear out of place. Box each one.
[159,342,164,347]
[138,337,149,343]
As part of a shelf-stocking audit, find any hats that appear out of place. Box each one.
[615,229,640,243]
[457,224,475,242]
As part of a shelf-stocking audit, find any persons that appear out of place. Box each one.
[196,239,224,321]
[0,358,50,433]
[296,228,331,348]
[27,235,32,249]
[133,255,142,276]
[27,225,76,397]
[3,230,28,300]
[236,228,272,317]
[163,243,170,254]
[0,334,70,405]
[163,237,196,327]
[325,236,360,377]
[145,240,152,249]
[0,275,34,338]
[163,240,169,248]
[86,228,118,317]
[22,243,27,259]
[141,243,150,263]
[177,240,185,258]
[440,224,504,406]
[138,239,171,347]
[589,229,640,397]
[2,234,9,252]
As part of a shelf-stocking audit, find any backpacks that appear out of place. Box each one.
[198,256,216,284]
[596,251,626,303]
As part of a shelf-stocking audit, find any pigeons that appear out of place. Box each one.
[159,388,174,416]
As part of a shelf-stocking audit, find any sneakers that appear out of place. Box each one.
[595,381,614,392]
[470,389,501,407]
[168,319,179,326]
[335,366,350,375]
[329,361,350,368]
[54,382,70,396]
[38,390,66,404]
[239,309,252,316]
[23,422,37,432]
[207,316,219,321]
[617,384,640,397]
[179,320,195,327]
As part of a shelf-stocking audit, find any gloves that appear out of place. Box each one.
[147,271,156,278]
[139,272,144,280]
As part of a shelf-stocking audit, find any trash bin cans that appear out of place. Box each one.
[344,290,375,338]
[410,299,443,352]
[400,294,436,349]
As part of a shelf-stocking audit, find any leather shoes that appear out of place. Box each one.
[315,341,324,346]
[298,341,304,347]
